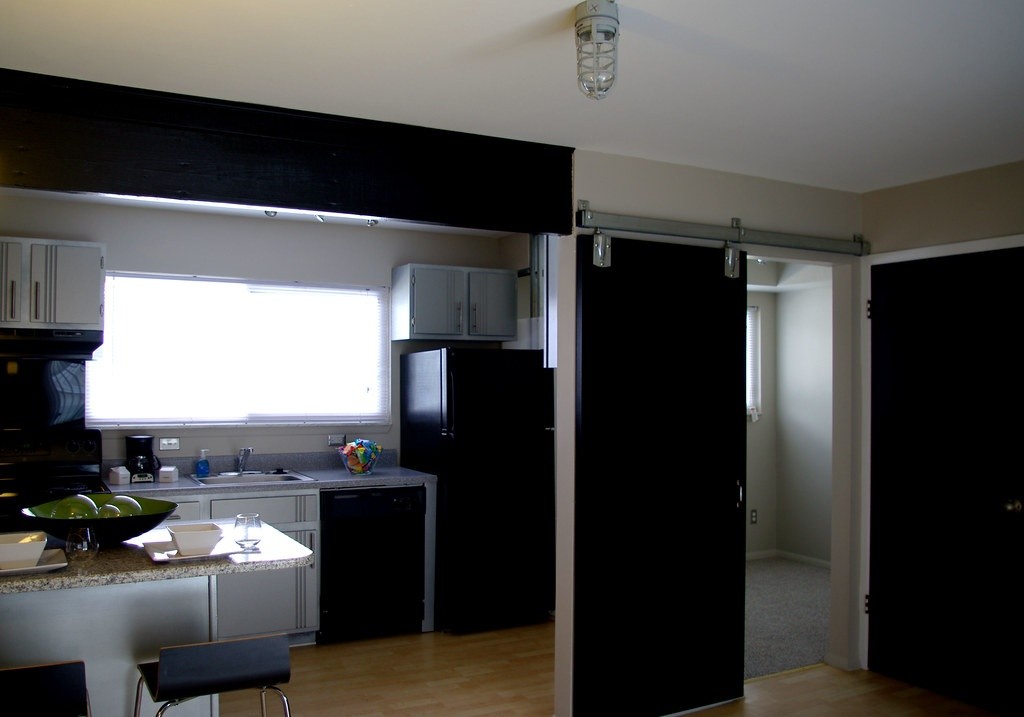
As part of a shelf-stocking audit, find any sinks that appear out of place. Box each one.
[195,473,302,485]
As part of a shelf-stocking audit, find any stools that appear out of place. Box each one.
[0,659,92,717]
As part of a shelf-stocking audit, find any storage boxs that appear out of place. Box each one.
[109,466,131,485]
[158,466,179,483]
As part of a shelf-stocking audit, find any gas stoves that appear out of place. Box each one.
[0,427,111,511]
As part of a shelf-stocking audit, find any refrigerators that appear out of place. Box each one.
[400,345,548,634]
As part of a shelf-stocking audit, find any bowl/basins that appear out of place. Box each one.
[167,522,223,556]
[0,531,47,568]
[22,493,178,548]
[339,453,380,475]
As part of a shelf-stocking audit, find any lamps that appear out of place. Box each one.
[573,0,620,102]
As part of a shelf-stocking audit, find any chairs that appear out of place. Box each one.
[133,631,291,717]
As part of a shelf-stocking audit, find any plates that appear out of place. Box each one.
[0,549,67,576]
[143,542,244,561]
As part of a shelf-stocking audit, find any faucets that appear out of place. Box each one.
[235,447,256,473]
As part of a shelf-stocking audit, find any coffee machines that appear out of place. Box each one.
[125,434,158,483]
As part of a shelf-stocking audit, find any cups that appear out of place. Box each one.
[233,513,263,548]
[65,525,100,562]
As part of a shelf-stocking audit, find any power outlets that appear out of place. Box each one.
[159,437,180,451]
[751,509,757,524]
[327,434,347,447]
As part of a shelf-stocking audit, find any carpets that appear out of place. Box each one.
[744,555,831,682]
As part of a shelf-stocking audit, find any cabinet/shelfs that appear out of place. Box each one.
[0,235,107,331]
[319,483,429,645]
[204,487,320,647]
[389,263,519,342]
[141,495,203,522]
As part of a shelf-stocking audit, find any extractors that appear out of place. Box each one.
[0,327,103,360]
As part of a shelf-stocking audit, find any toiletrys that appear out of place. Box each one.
[197,448,211,477]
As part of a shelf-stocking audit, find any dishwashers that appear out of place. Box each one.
[321,486,425,645]
